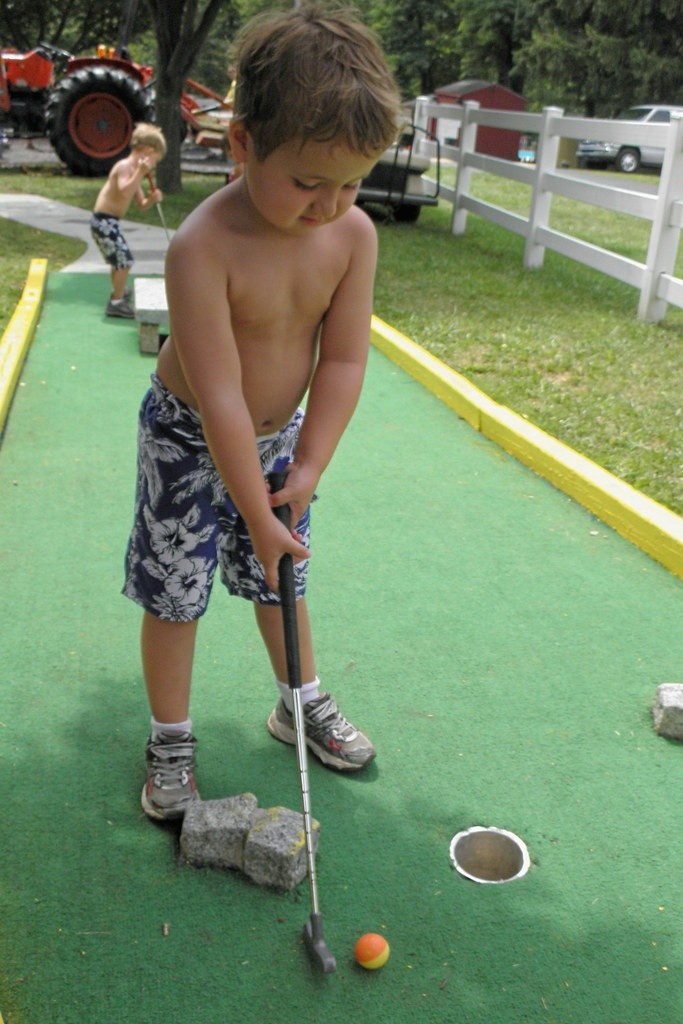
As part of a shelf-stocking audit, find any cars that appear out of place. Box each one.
[575,104,683,173]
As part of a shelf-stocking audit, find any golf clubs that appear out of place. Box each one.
[147,168,170,246]
[271,469,339,978]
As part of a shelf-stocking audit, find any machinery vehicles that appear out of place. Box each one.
[0,42,234,178]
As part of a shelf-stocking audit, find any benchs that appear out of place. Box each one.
[133,273,170,354]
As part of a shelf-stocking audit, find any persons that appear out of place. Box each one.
[89,119,167,321]
[115,4,404,822]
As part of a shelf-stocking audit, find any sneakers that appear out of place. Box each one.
[106,300,135,318]
[141,728,204,821]
[111,290,132,301]
[268,691,376,772]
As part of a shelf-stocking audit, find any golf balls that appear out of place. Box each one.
[354,934,392,973]
